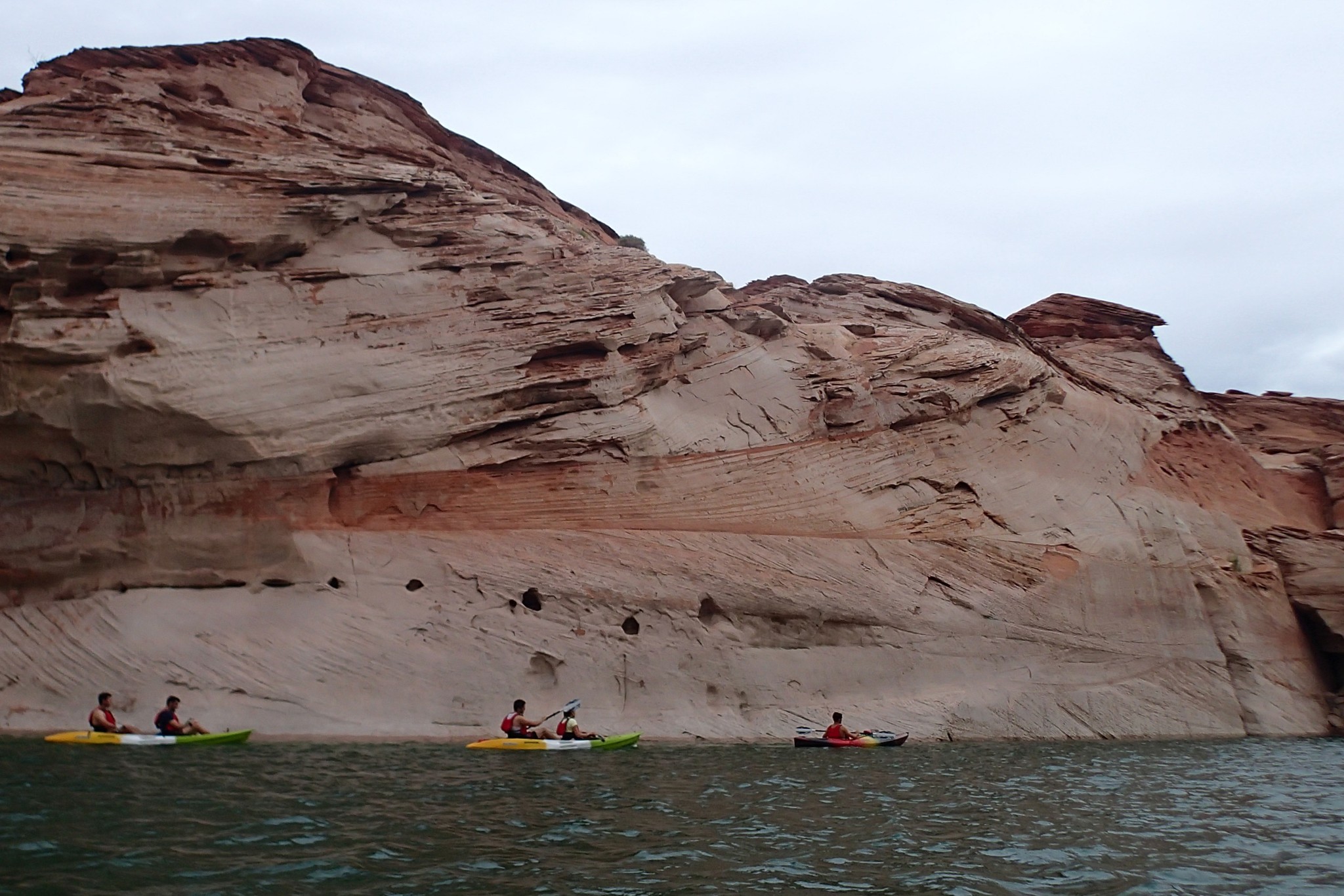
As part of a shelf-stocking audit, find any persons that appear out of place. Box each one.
[501,700,561,740]
[556,706,596,740]
[89,692,142,735]
[154,696,229,735]
[823,712,860,739]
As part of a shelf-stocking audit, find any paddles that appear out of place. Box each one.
[795,726,896,740]
[527,698,581,730]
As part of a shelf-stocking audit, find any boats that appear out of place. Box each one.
[793,730,909,747]
[43,726,256,745]
[465,731,643,750]
